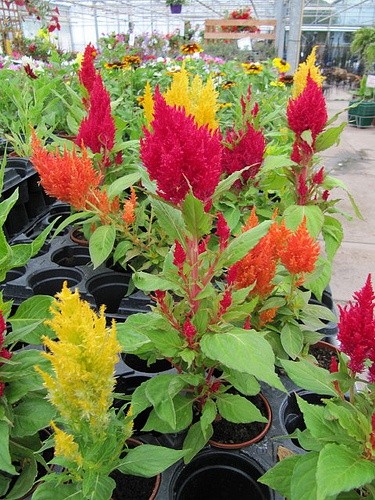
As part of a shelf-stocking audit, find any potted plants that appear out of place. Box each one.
[164,0,187,15]
[345,26,374,128]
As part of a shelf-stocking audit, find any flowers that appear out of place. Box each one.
[94,35,301,118]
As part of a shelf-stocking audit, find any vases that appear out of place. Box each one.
[0,149,361,499]
